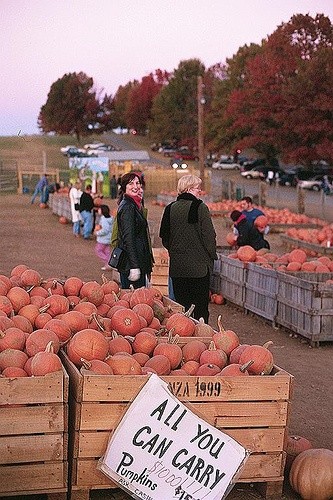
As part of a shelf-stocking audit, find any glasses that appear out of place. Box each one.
[192,186,201,190]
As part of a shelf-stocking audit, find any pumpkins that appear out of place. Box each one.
[251,203,330,228]
[40,187,69,224]
[287,435,312,469]
[197,191,206,196]
[152,190,178,206]
[289,448,333,500]
[205,199,244,218]
[228,245,333,285]
[226,233,238,246]
[0,264,273,377]
[154,249,169,267]
[93,197,117,231]
[285,223,333,247]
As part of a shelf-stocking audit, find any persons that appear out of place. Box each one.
[159,175,218,326]
[42,181,65,208]
[231,209,270,251]
[31,173,49,204]
[240,197,268,238]
[79,183,95,239]
[69,182,84,238]
[94,205,115,270]
[118,173,123,190]
[110,175,117,199]
[109,173,155,288]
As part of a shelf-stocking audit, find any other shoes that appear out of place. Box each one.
[101,267,107,271]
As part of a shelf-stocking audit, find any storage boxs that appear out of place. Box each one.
[156,194,178,208]
[222,217,324,233]
[48,193,72,223]
[281,234,332,258]
[150,247,171,297]
[0,294,293,500]
[211,247,333,345]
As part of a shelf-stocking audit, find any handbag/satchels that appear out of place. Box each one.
[108,248,127,272]
[74,203,80,210]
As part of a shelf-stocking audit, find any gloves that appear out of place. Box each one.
[128,268,141,281]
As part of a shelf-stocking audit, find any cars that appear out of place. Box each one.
[151,140,333,196]
[60,139,119,159]
